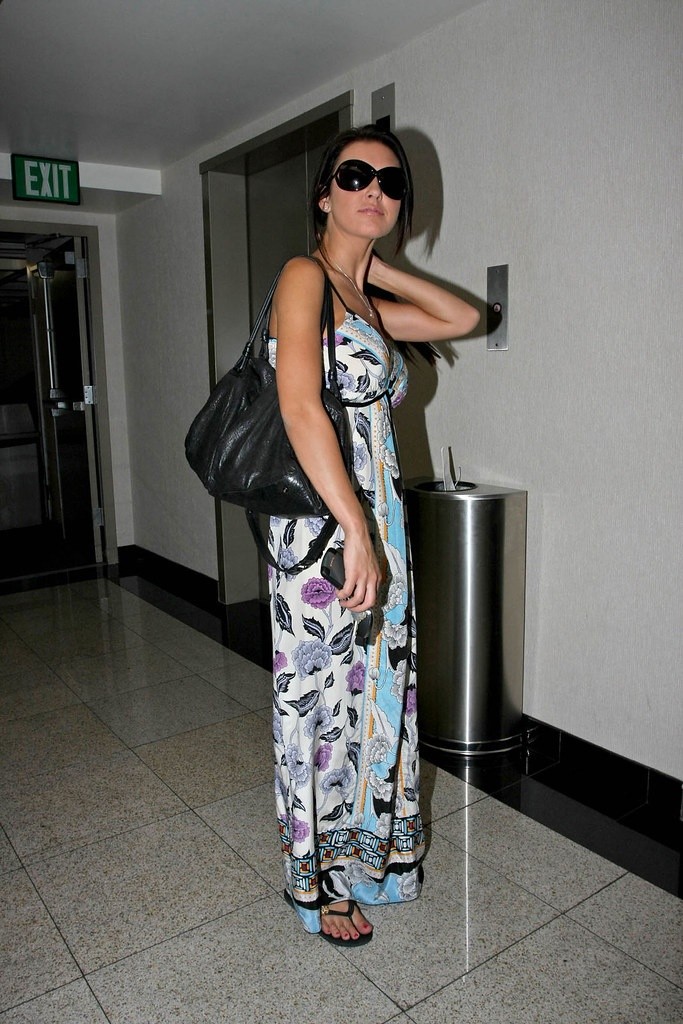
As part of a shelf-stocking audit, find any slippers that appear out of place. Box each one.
[284,888,373,947]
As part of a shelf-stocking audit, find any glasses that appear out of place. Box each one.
[329,159,408,200]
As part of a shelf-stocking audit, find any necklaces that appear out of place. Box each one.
[328,254,375,318]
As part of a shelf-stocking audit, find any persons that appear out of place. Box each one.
[266,122,480,949]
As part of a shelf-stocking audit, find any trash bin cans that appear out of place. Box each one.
[397,480,530,777]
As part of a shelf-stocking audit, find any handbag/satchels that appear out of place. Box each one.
[184,253,353,575]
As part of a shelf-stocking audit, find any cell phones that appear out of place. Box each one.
[320,547,364,605]
